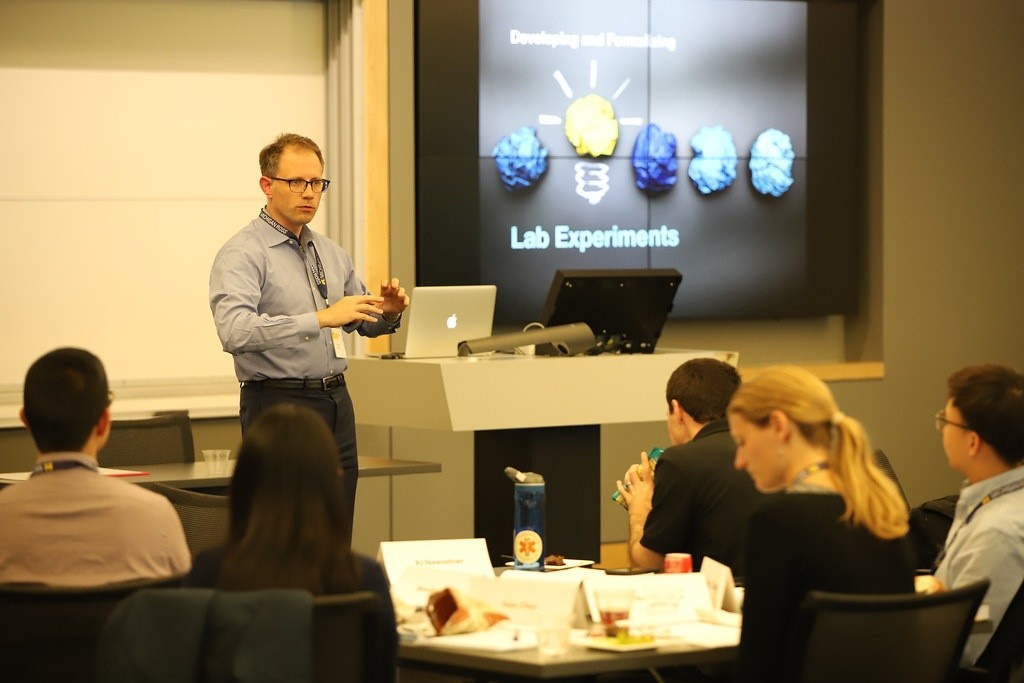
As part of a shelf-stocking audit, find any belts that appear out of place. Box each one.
[244,373,347,392]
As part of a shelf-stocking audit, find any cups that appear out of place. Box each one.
[202,449,231,476]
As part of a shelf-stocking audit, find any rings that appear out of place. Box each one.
[623,482,632,490]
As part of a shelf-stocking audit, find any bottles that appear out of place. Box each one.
[505,468,547,572]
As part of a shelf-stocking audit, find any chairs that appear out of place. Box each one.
[137,588,378,683]
[0,575,186,683]
[149,481,229,559]
[787,577,991,683]
[97,416,195,468]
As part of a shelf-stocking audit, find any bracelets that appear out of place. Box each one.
[382,311,402,320]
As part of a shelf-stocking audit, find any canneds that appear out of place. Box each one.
[664,552,693,574]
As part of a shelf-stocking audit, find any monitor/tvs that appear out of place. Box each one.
[533,268,682,357]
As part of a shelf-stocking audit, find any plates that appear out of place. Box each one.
[505,559,595,570]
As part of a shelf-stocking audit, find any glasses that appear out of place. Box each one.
[98,391,116,420]
[935,409,972,433]
[271,176,331,194]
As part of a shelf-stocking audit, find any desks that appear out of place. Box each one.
[397,568,744,683]
[0,458,441,491]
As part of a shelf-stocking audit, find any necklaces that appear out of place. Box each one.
[788,460,831,490]
[31,460,94,475]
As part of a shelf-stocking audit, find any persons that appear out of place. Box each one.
[188,404,399,683]
[616,358,767,587]
[727,365,916,683]
[211,134,409,552]
[934,363,1024,670]
[0,346,191,587]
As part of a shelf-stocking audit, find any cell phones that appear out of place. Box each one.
[606,567,660,575]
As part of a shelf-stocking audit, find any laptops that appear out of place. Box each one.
[365,285,496,359]
